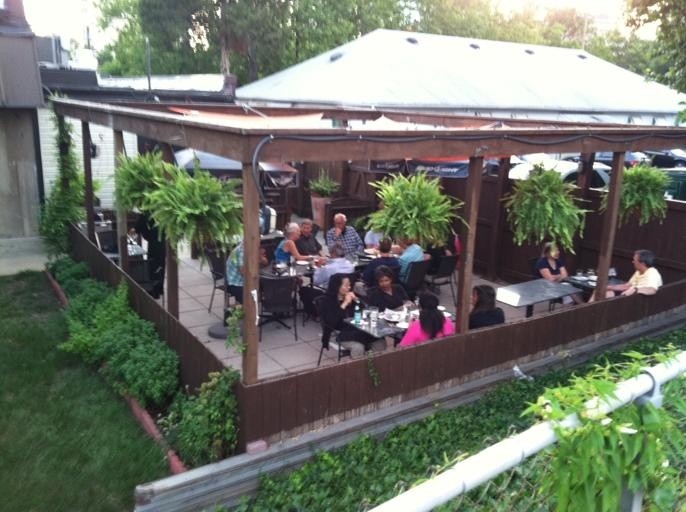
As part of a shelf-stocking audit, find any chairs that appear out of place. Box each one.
[428,256,459,306]
[312,294,341,366]
[203,249,236,314]
[259,275,299,342]
[301,272,357,328]
[527,256,562,312]
[390,265,402,280]
[403,260,429,300]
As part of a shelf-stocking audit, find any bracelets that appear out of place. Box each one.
[634,287,638,294]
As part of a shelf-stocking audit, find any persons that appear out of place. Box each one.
[399,293,455,349]
[299,244,354,320]
[469,285,505,330]
[226,238,269,304]
[327,214,364,256]
[297,219,327,260]
[273,223,314,266]
[589,250,663,304]
[368,265,410,350]
[396,235,424,286]
[354,236,401,308]
[423,239,452,283]
[364,231,391,249]
[318,273,387,359]
[532,242,583,304]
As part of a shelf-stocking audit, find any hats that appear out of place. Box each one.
[419,292,440,309]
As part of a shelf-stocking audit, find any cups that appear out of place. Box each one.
[371,317,377,327]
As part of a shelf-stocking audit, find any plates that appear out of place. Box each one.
[437,305,446,311]
[397,322,410,328]
[443,311,452,318]
[383,314,402,322]
[296,260,309,266]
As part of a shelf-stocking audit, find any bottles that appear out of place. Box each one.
[354,306,360,325]
